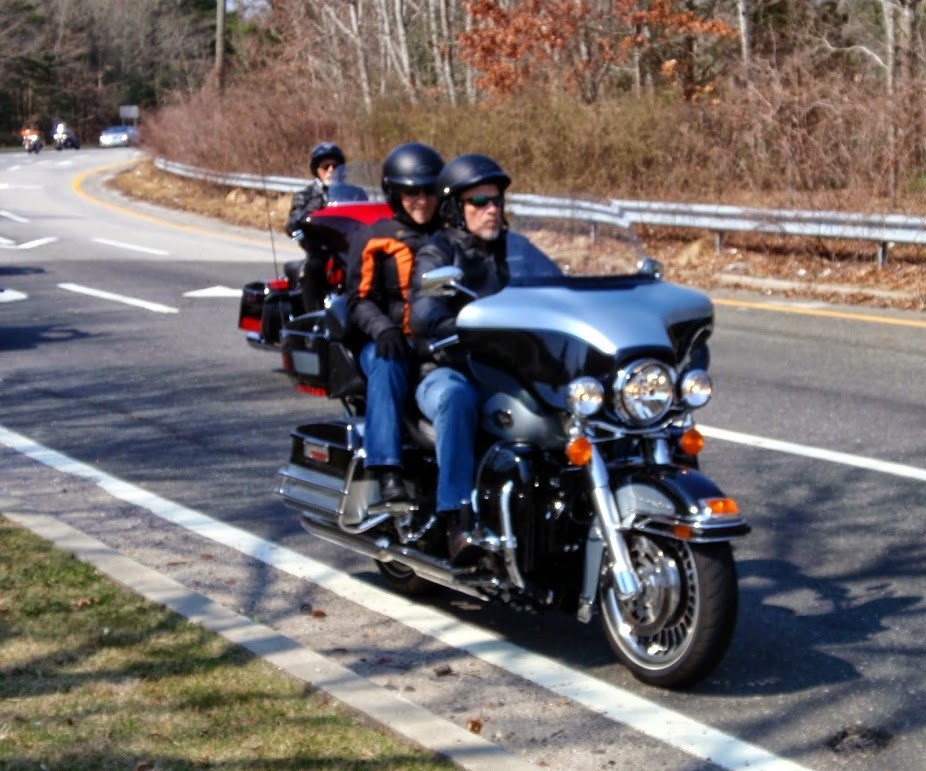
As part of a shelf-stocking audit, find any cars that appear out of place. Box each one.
[99,126,139,148]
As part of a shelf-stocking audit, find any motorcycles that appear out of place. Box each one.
[23,132,42,154]
[53,125,68,150]
[242,215,753,690]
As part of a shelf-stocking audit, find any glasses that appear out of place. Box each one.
[460,195,504,208]
[317,162,343,171]
[402,188,438,198]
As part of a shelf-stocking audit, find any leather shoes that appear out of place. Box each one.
[376,469,409,502]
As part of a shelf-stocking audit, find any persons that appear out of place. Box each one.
[347,142,445,535]
[409,153,564,568]
[284,142,368,281]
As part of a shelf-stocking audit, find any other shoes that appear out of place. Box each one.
[448,510,485,566]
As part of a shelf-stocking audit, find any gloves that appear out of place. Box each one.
[375,329,412,361]
[438,319,474,355]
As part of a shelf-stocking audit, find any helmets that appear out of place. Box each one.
[310,144,345,177]
[381,142,442,191]
[436,154,511,201]
